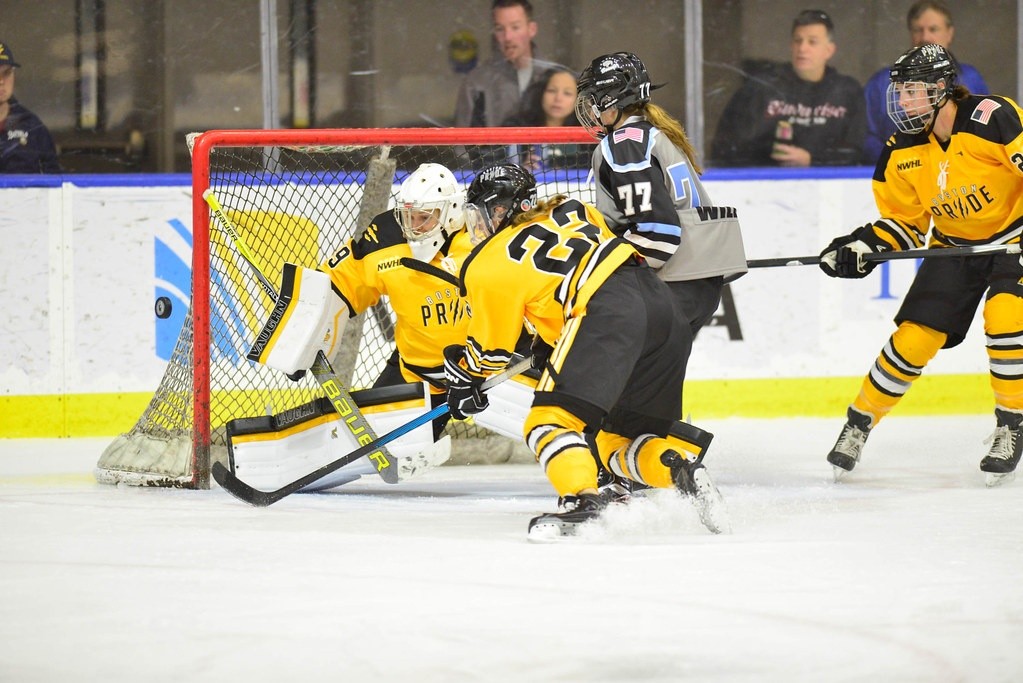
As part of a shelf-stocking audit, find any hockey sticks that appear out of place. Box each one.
[748,241,1023,269]
[200,185,454,486]
[211,356,531,508]
[400,255,460,289]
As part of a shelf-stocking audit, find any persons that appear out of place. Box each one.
[445,0,604,169]
[0,44,59,173]
[249,163,730,544]
[819,43,1023,487]
[573,52,746,507]
[862,3,991,165]
[709,11,870,168]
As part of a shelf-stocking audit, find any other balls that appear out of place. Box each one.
[154,297,172,319]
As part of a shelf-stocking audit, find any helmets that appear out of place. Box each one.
[465,163,538,247]
[574,51,651,138]
[885,44,957,136]
[396,163,466,264]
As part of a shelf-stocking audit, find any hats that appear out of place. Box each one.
[0,39,19,65]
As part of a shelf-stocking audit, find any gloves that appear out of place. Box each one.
[819,223,896,279]
[441,343,493,419]
[529,330,555,371]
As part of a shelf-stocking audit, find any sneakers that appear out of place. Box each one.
[521,493,626,541]
[667,448,733,531]
[977,410,1023,485]
[825,408,874,484]
